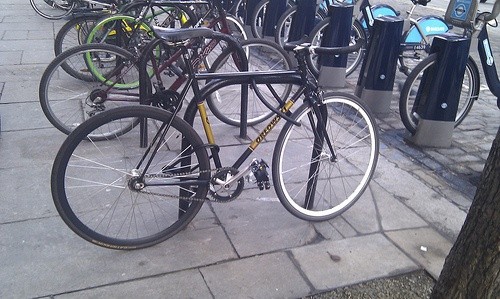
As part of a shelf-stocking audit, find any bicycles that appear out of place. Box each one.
[50,35,380,251]
[28,0,500,141]
